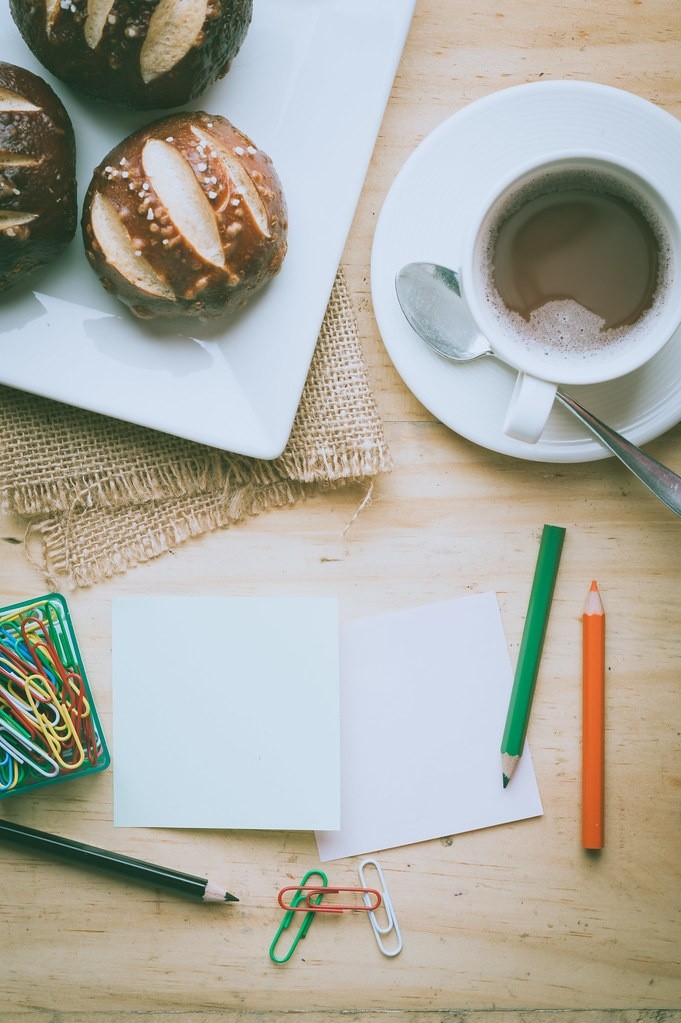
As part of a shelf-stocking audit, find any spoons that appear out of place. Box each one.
[396,256,681,529]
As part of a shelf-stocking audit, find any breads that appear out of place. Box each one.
[1,0,290,320]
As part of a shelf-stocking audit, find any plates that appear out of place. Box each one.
[0,0,422,463]
[368,75,681,463]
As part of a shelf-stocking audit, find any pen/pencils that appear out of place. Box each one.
[0,819,240,902]
[583,580,606,850]
[500,525,566,789]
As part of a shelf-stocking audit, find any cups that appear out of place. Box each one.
[457,154,681,385]
